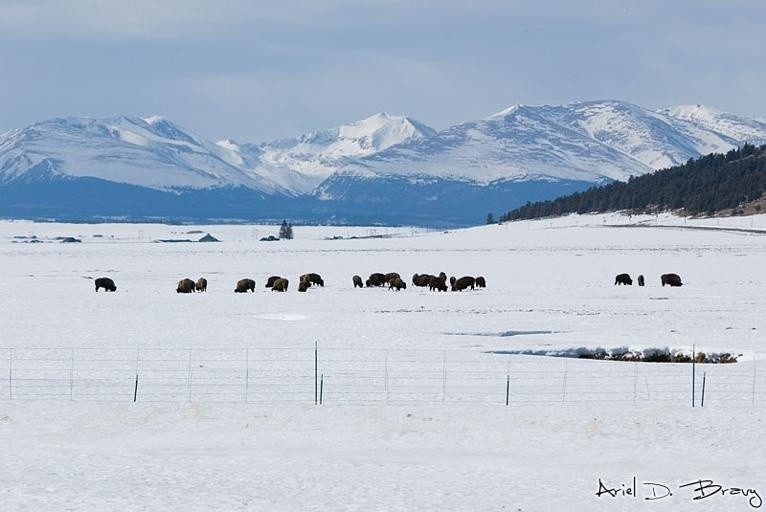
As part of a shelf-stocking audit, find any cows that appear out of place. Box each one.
[661,273,683,287]
[366,273,406,290]
[176,277,207,293]
[265,276,288,292]
[638,276,644,286]
[235,279,255,293]
[615,273,633,285]
[94,277,117,293]
[353,275,363,288]
[413,272,486,291]
[300,273,324,292]
